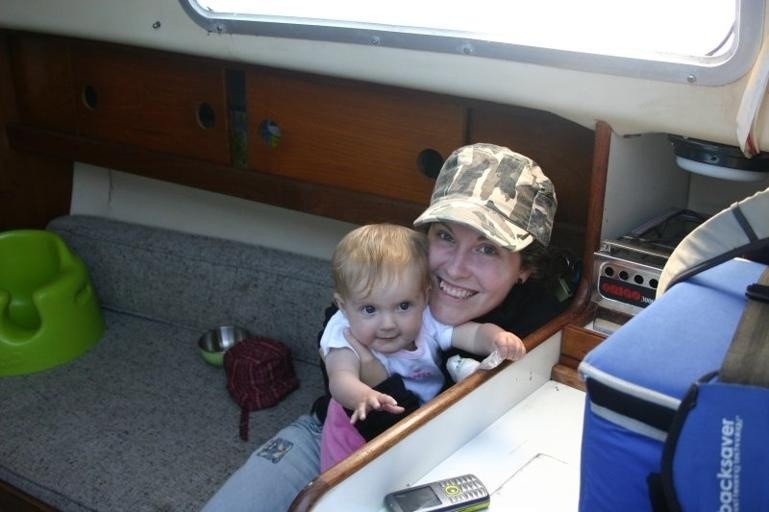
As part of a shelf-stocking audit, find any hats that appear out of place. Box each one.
[412,142,558,254]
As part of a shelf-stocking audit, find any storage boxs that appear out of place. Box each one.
[574,251,769,512]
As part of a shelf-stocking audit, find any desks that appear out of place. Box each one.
[286,120,769,512]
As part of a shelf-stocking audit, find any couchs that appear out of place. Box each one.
[0,212,344,511]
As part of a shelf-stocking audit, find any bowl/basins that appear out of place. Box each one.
[197,325,248,368]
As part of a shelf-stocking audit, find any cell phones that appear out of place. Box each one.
[384,472,490,512]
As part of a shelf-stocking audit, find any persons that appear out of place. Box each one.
[314,142,559,429]
[318,224,525,472]
